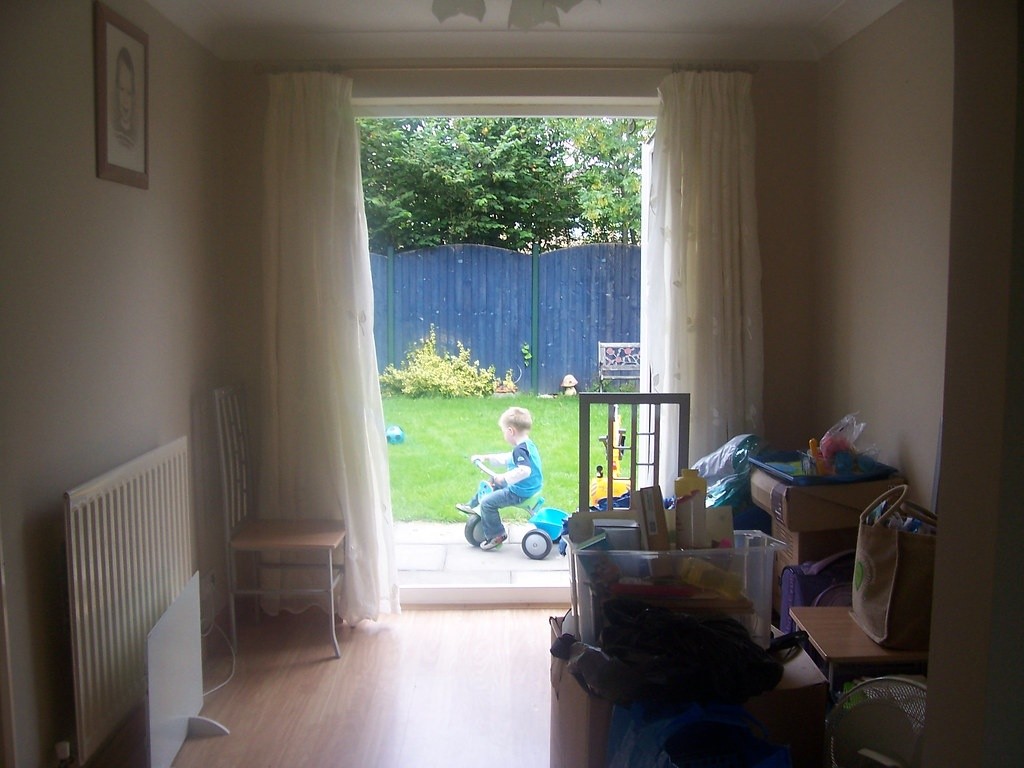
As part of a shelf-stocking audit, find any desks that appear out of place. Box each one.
[788,607,929,681]
[743,450,907,628]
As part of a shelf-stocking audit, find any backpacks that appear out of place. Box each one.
[811,583,894,709]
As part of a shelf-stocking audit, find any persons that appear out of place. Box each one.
[455,407,543,550]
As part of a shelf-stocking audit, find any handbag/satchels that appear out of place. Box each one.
[780,549,857,659]
[849,484,934,651]
[606,704,790,768]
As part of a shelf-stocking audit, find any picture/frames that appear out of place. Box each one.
[92,0,149,189]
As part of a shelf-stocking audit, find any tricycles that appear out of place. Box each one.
[463,457,571,560]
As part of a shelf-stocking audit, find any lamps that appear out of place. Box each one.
[432,0,584,33]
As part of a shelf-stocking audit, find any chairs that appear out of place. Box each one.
[215,389,358,658]
[580,392,692,513]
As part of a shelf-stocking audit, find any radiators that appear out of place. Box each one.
[61,439,194,767]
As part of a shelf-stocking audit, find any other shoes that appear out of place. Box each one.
[457,504,475,514]
[480,533,507,550]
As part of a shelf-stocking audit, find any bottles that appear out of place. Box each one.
[674,468,706,558]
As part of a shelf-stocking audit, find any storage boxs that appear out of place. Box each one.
[560,517,787,651]
[549,617,828,768]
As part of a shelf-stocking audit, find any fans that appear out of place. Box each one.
[823,676,927,768]
